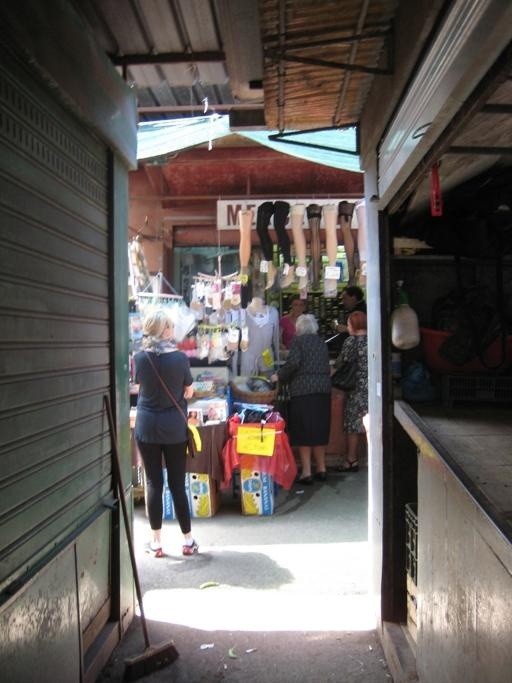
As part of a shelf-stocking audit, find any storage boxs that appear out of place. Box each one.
[144,463,221,518]
[241,455,274,516]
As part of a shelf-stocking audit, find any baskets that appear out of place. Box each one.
[231,382,275,404]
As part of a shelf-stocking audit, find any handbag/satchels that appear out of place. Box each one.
[187,428,196,457]
[332,360,358,390]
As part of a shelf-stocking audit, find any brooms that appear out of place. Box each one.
[104,395,180,679]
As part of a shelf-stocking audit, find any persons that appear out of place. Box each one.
[204,403,224,422]
[324,286,367,360]
[185,408,203,427]
[278,313,333,487]
[338,202,358,289]
[230,297,281,384]
[133,308,199,556]
[290,202,310,291]
[307,203,323,289]
[235,207,256,311]
[328,311,368,473]
[257,200,295,291]
[353,201,368,288]
[320,205,338,300]
[277,294,307,348]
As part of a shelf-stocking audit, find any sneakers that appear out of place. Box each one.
[183,540,198,554]
[145,542,161,557]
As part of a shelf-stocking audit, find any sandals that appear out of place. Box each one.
[335,459,358,472]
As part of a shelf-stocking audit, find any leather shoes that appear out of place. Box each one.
[315,472,326,481]
[297,476,312,484]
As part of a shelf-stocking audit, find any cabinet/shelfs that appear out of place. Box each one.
[281,290,352,359]
